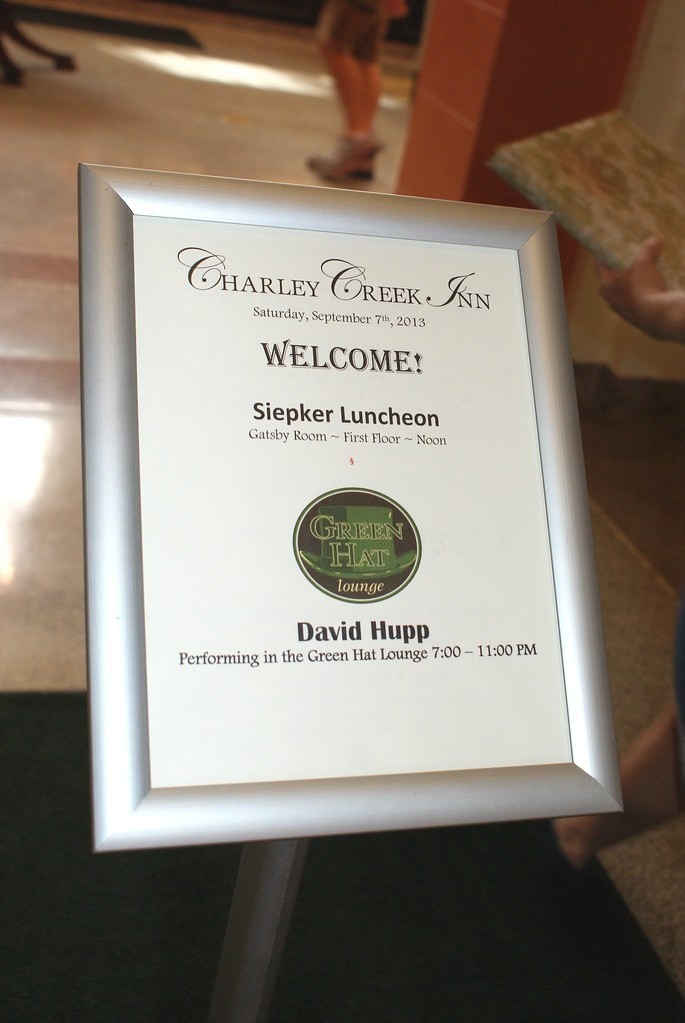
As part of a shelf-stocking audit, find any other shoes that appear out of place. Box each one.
[309,134,384,185]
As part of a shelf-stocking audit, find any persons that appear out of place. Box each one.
[554,235,685,855]
[307,0,408,180]
